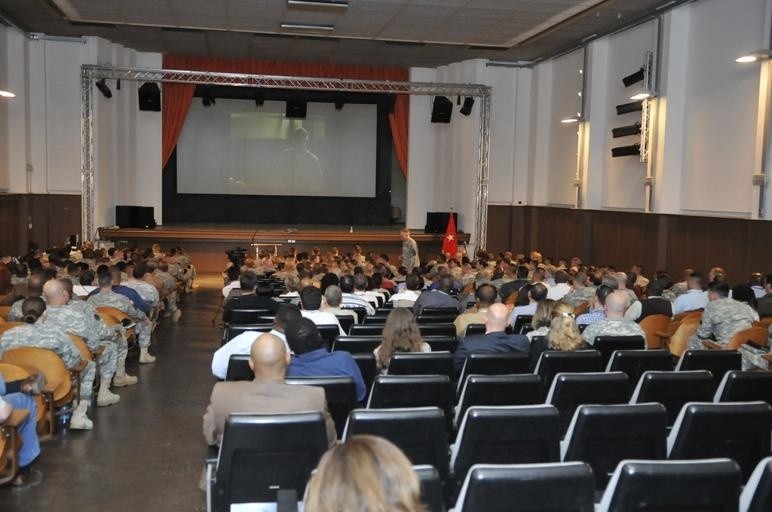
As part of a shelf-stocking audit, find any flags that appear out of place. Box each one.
[441,211,457,258]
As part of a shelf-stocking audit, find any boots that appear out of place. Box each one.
[70,280,199,430]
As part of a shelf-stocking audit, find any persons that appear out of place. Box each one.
[285,317,367,402]
[203,333,338,450]
[303,434,429,512]
[400,228,420,273]
[1,241,196,493]
[280,126,320,185]
[213,245,771,381]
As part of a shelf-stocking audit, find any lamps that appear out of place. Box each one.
[629,91,657,100]
[560,114,583,123]
[281,24,334,31]
[735,48,769,65]
[288,0,349,8]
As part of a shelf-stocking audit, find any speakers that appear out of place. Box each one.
[285,98,307,119]
[115,205,154,228]
[424,212,458,233]
[431,96,453,122]
[138,83,160,112]
